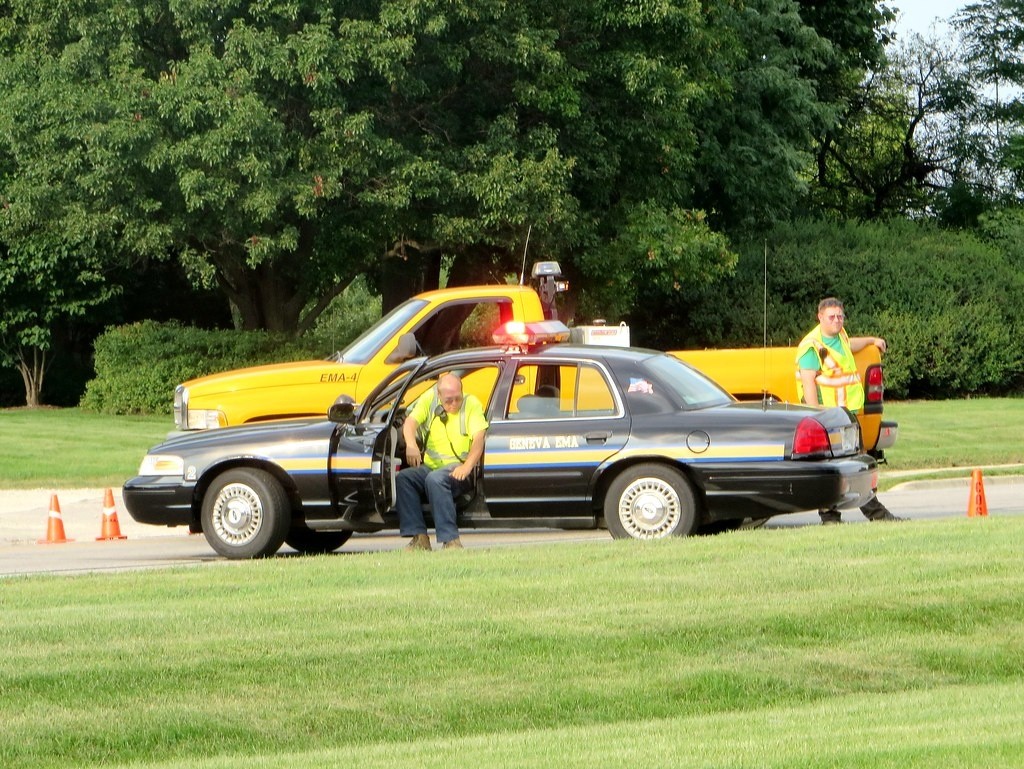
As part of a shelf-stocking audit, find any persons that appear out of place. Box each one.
[394,372,490,552]
[794,298,908,525]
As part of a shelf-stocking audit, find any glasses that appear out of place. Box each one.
[821,313,844,320]
[439,391,463,403]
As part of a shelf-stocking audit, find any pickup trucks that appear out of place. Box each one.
[167,282,900,473]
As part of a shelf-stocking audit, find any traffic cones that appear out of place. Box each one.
[94,486,130,540]
[37,492,77,545]
[968,468,988,516]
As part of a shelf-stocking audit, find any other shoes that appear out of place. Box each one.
[405,534,432,551]
[821,512,845,524]
[870,512,911,521]
[442,537,462,549]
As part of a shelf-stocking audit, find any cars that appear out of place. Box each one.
[120,344,880,550]
